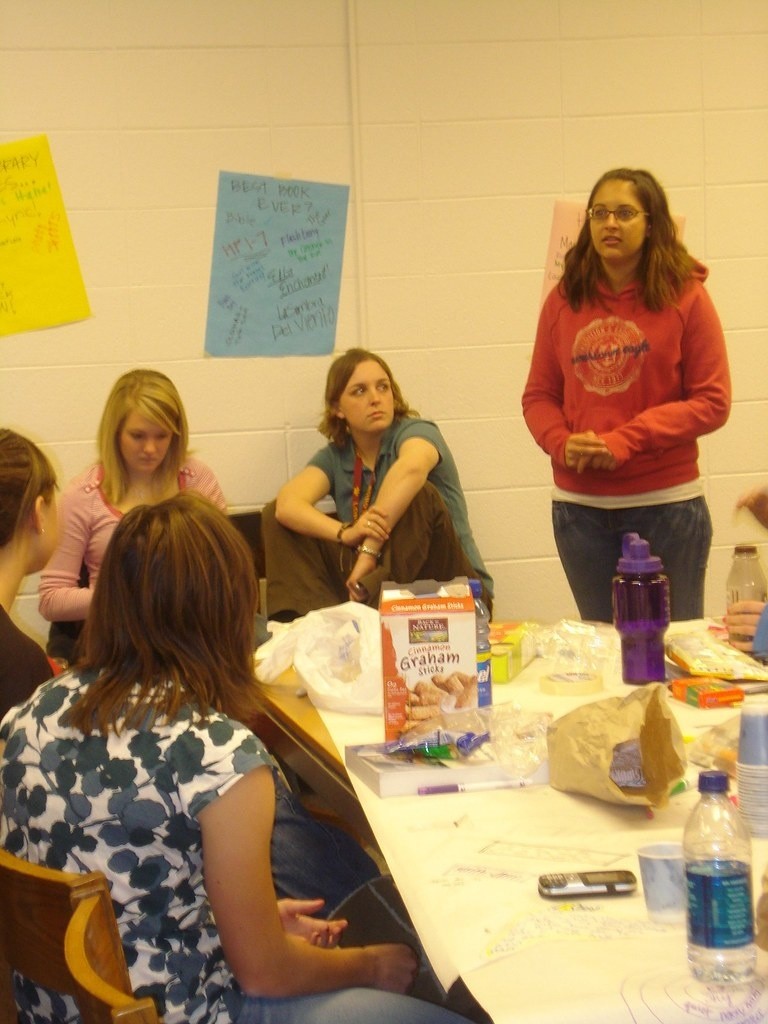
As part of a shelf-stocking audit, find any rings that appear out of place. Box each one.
[354,583,364,594]
[367,520,370,528]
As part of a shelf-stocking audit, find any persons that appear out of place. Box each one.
[0,498,447,1024]
[0,370,227,721]
[521,169,731,621]
[275,349,494,623]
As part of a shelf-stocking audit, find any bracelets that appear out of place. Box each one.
[355,544,382,557]
[337,522,353,548]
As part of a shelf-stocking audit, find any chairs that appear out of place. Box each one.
[63,885,164,1024]
[227,511,267,612]
[0,847,110,1024]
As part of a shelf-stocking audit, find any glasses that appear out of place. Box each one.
[584,204,650,221]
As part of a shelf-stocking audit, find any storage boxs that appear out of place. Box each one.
[379,579,482,740]
[669,674,744,710]
[488,621,540,684]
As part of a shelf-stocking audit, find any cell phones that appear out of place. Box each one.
[539,871,637,899]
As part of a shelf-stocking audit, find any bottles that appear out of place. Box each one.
[683,771,759,985]
[728,545,767,667]
[612,532,670,686]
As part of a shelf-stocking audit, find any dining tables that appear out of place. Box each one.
[234,615,768,1024]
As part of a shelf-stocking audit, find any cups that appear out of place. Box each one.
[637,843,688,924]
[737,705,767,837]
